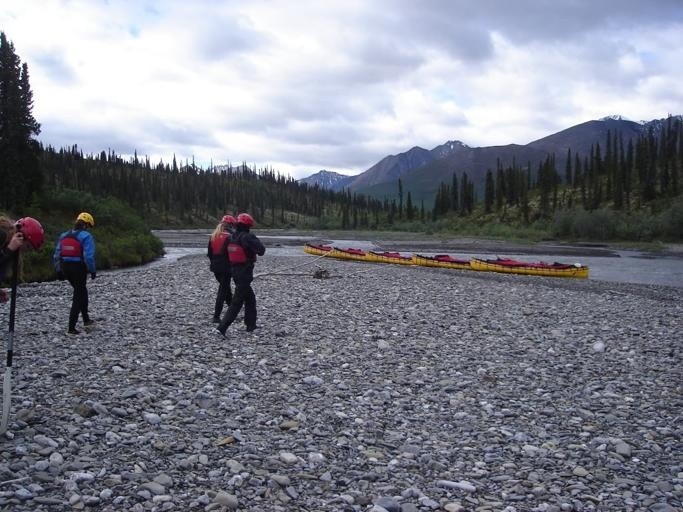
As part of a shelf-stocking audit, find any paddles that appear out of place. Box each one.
[0,223,22,439]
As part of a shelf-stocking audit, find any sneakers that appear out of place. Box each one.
[68,329,80,334]
[83,320,93,325]
[215,328,230,340]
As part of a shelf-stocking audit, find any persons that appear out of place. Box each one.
[0,217,45,303]
[206,215,236,323]
[53,213,96,334]
[216,213,265,339]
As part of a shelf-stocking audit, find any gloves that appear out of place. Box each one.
[91,272,95,279]
[57,271,64,281]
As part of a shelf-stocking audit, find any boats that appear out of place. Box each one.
[368,250,410,265]
[303,243,331,257]
[331,246,367,261]
[468,257,589,280]
[411,252,470,269]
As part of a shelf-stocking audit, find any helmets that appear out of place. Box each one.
[15,216,45,251]
[77,212,94,227]
[221,215,237,225]
[236,212,253,225]
